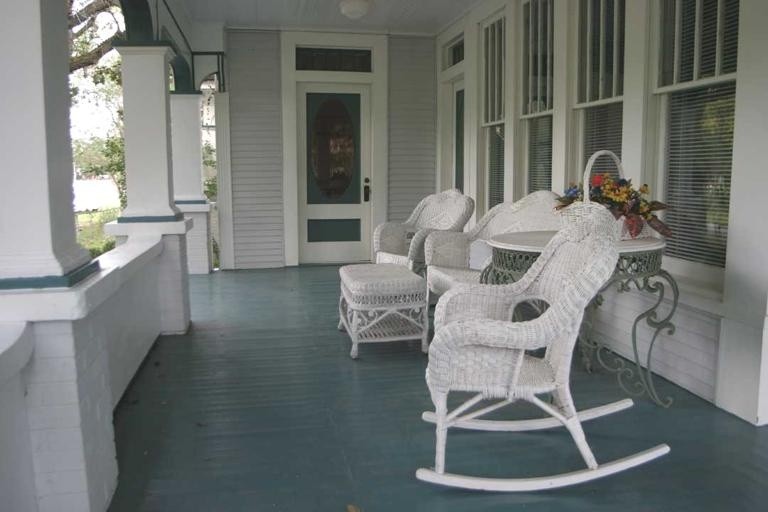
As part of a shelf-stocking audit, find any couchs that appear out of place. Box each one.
[373,189,475,306]
[424,190,565,311]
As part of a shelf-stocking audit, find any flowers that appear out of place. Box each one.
[554,150,675,241]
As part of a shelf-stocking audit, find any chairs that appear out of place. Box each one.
[414,209,671,493]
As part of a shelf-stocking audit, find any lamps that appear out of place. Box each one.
[340,1,371,20]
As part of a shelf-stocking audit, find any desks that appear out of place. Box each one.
[480,228,679,410]
